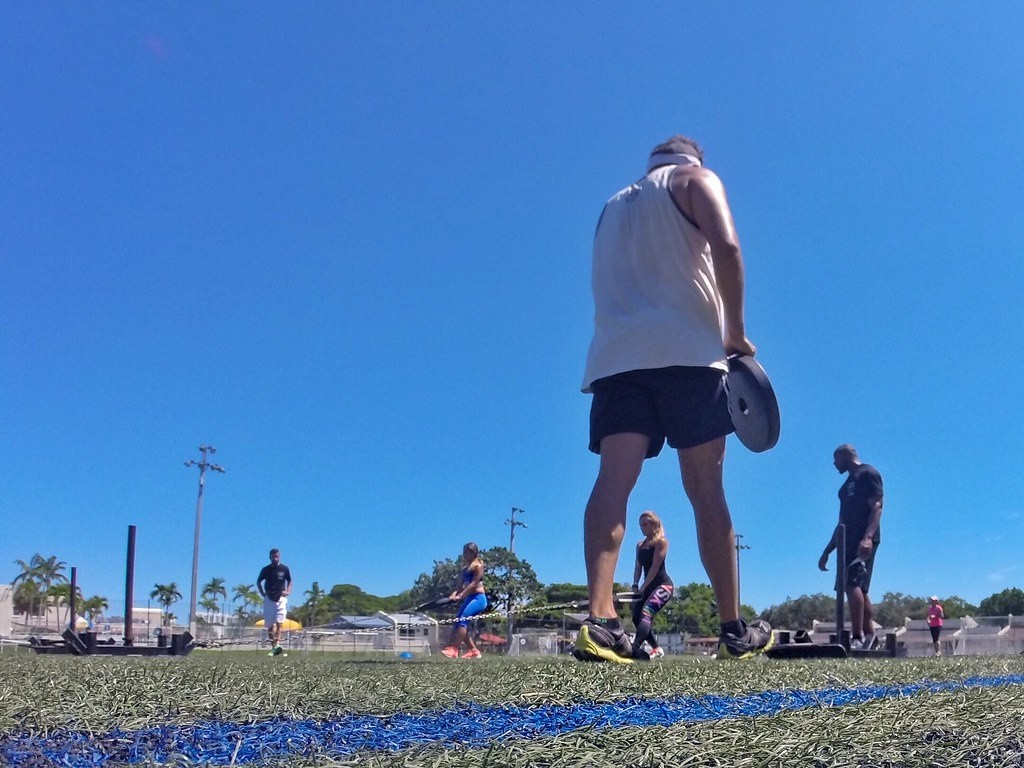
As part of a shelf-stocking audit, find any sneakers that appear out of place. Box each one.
[649,648,664,659]
[862,635,880,650]
[461,649,482,659]
[573,617,650,664]
[850,639,863,648]
[441,645,459,659]
[268,652,274,657]
[716,619,775,661]
[272,646,282,655]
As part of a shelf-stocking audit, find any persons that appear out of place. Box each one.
[818,445,884,650]
[927,595,944,657]
[573,134,775,662]
[632,512,674,659]
[257,549,292,656]
[440,542,487,660]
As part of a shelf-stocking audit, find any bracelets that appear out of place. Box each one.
[632,584,638,589]
[458,593,463,598]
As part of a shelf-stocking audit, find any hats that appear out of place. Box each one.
[928,596,938,601]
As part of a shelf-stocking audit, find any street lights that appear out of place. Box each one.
[734,533,751,619]
[183,443,227,646]
[505,507,530,656]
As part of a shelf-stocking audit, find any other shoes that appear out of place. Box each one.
[935,651,941,657]
